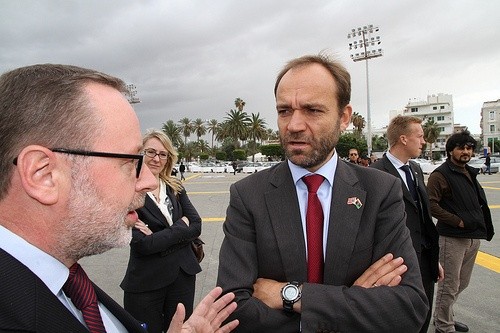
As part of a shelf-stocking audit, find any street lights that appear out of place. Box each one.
[346,23,384,166]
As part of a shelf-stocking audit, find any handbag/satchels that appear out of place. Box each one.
[191,238,205,263]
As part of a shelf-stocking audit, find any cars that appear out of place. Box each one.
[171,160,283,173]
[409,156,500,176]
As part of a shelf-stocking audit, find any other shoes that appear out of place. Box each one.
[433,321,469,333]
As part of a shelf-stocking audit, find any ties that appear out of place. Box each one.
[300,175,326,283]
[401,165,424,227]
[64,262,107,333]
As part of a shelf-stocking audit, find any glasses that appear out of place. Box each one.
[455,140,472,148]
[49,147,145,179]
[144,149,168,160]
[350,153,357,156]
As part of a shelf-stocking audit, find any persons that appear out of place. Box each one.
[346,148,379,166]
[214,56,429,333]
[120,129,202,333]
[367,115,445,333]
[483,153,492,175]
[426,131,495,333]
[179,162,185,180]
[0,63,240,333]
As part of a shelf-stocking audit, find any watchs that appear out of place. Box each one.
[280,281,303,312]
